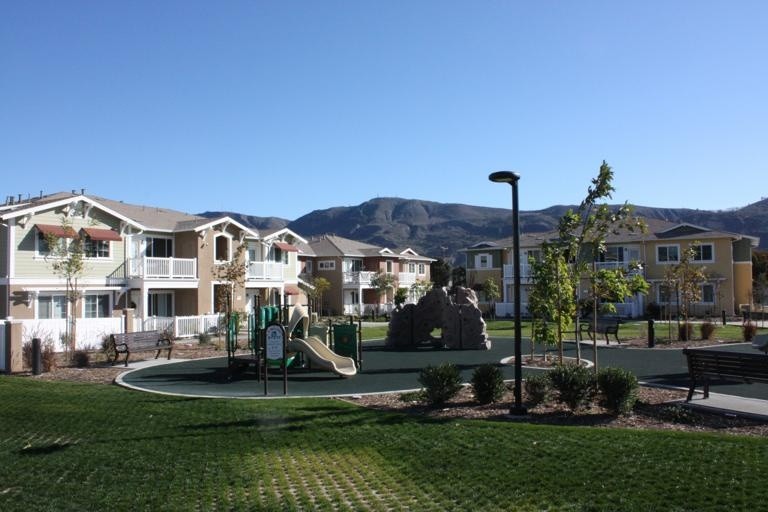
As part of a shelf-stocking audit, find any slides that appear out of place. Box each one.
[288,306,357,377]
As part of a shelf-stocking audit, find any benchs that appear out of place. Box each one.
[681,348,768,402]
[578,318,622,345]
[110,330,174,367]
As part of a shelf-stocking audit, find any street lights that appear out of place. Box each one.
[487,171,527,415]
